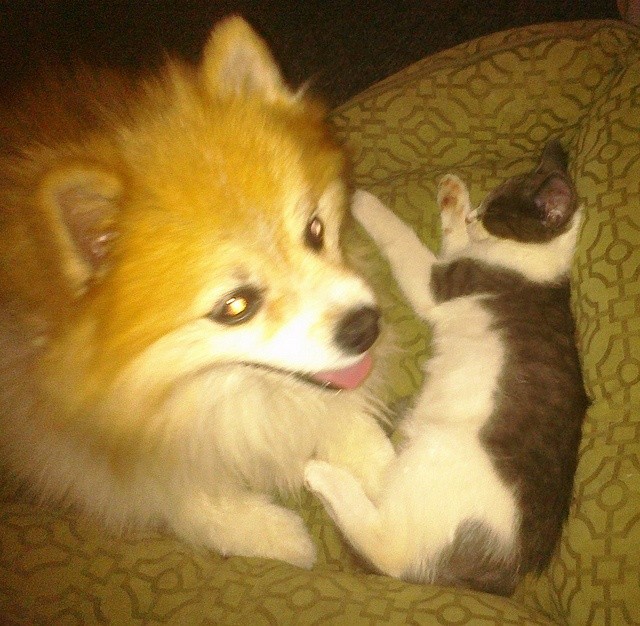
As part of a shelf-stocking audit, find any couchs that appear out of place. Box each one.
[2,17,640,626]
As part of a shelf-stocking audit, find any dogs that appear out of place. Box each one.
[0,16,380,571]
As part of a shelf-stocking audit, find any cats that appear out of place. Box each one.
[304,138,588,592]
